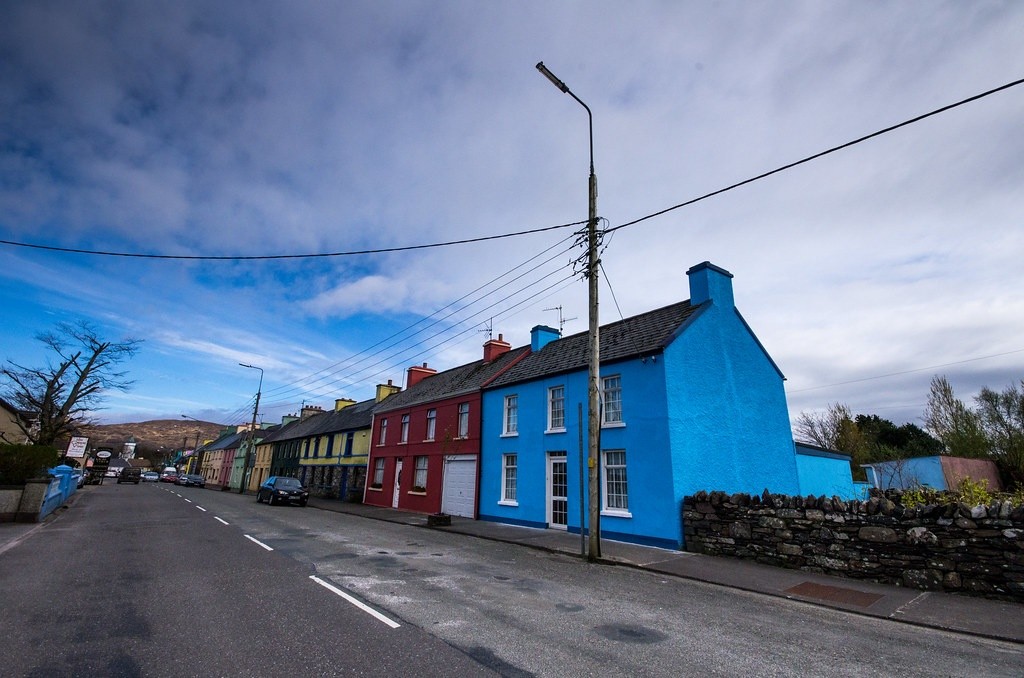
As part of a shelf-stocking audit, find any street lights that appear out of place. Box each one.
[535,61,602,559]
[239,362,264,494]
[181,414,201,475]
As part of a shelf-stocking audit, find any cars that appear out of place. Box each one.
[140,472,146,480]
[72,468,85,489]
[186,475,207,489]
[256,477,310,507]
[142,472,159,482]
[174,473,190,486]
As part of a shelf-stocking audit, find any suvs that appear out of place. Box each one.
[117,467,141,484]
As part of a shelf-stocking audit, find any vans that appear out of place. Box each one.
[160,467,176,482]
